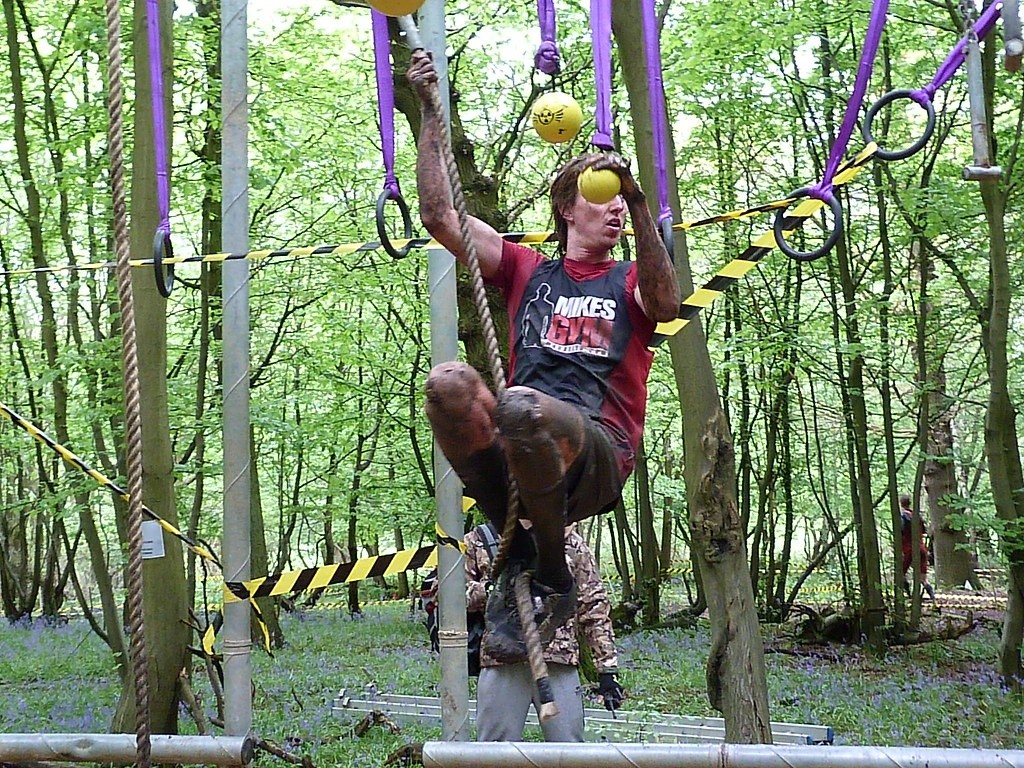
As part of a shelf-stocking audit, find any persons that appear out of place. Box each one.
[900,497,936,604]
[420,566,481,678]
[464,520,622,742]
[406,51,682,664]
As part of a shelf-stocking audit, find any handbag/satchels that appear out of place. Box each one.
[422,517,506,677]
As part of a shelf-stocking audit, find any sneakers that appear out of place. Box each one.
[484,538,577,665]
